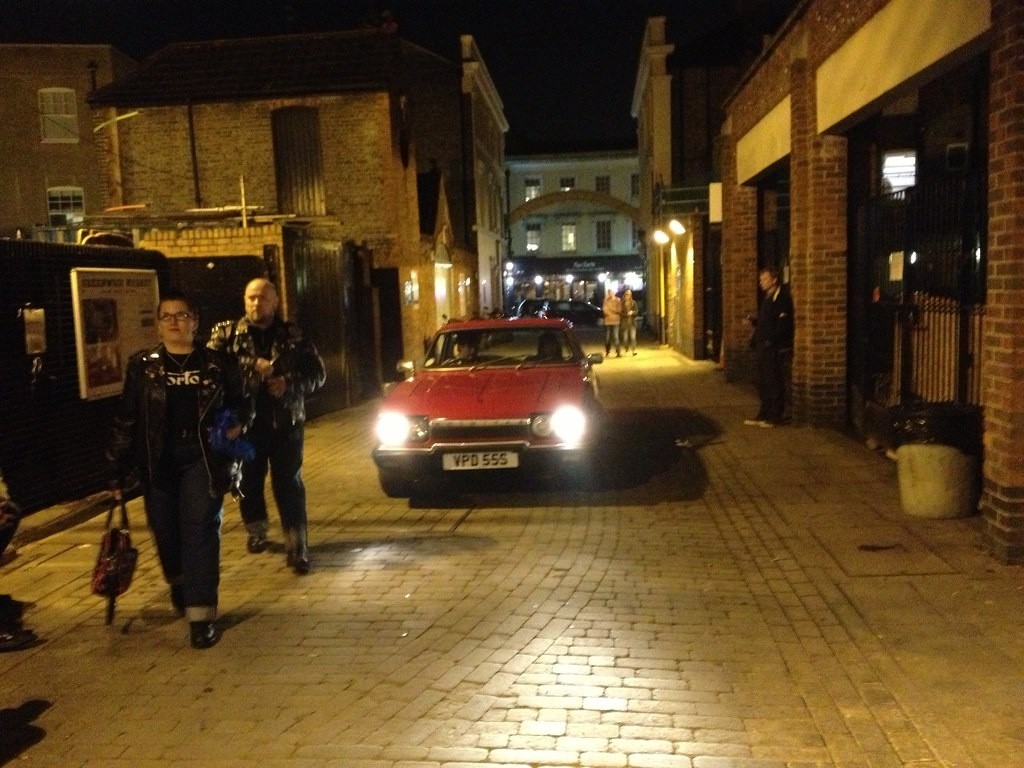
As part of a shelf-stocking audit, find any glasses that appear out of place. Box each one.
[159,311,195,322]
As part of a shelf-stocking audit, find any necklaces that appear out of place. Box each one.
[163,348,194,374]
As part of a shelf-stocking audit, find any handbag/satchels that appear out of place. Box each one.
[93,499,138,598]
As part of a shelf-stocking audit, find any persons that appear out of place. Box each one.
[491,283,553,319]
[106,291,256,648]
[741,268,794,428]
[207,278,327,575]
[602,289,623,357]
[620,290,639,356]
[0,474,36,649]
[523,333,565,364]
[442,335,481,368]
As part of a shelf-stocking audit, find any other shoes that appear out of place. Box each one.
[744,418,764,426]
[617,354,623,357]
[246,528,267,553]
[189,618,218,649]
[0,634,16,648]
[633,351,637,356]
[284,541,311,574]
[865,437,878,450]
[761,421,777,429]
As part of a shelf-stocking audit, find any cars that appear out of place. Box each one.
[372,318,604,495]
[517,299,605,326]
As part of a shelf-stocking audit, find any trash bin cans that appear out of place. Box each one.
[885,401,984,517]
